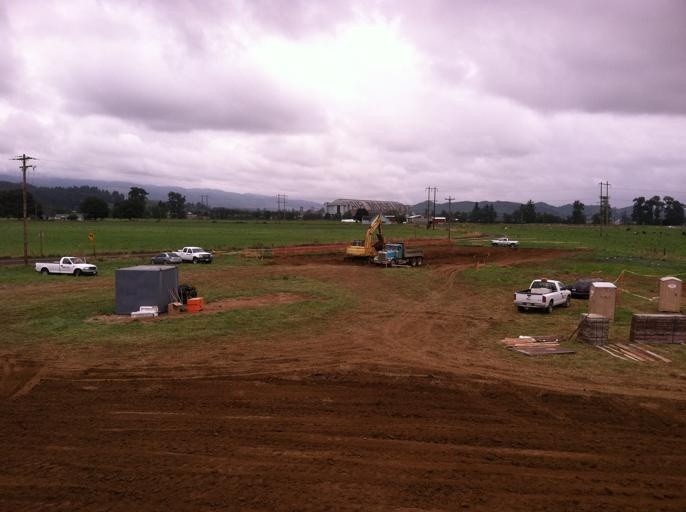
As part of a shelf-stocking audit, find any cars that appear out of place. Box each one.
[567,278,607,299]
[151,252,182,265]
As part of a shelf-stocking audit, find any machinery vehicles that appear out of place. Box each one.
[343,214,385,264]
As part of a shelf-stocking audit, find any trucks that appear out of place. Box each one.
[373,241,424,269]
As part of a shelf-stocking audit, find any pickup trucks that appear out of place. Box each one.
[491,237,520,248]
[172,246,213,264]
[35,256,97,277]
[513,278,572,314]
